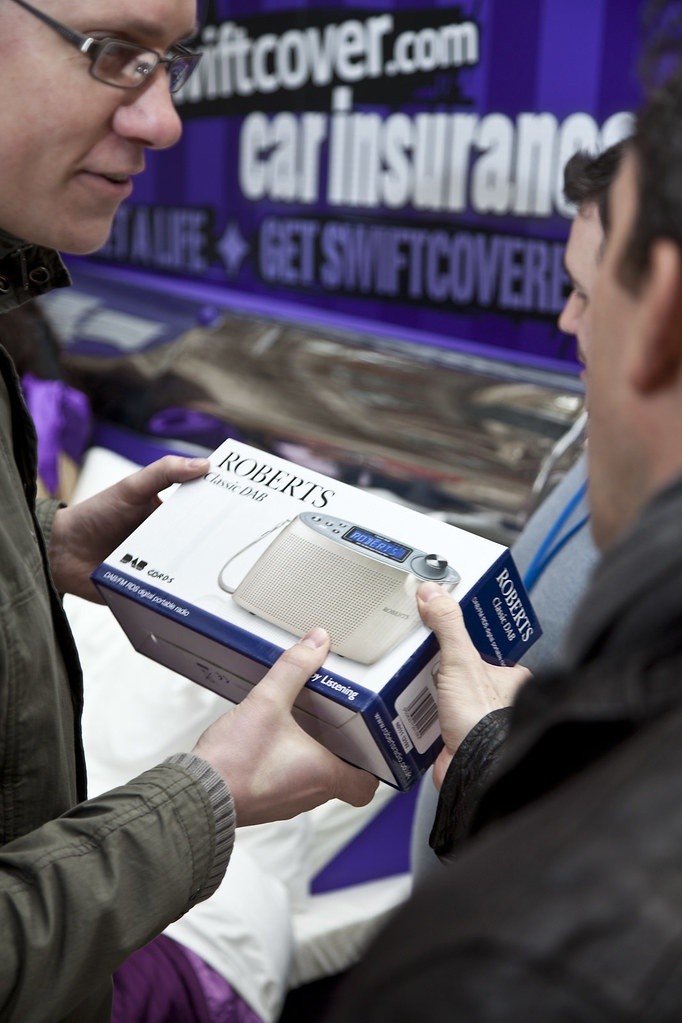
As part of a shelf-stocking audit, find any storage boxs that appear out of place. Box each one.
[89,438,543,789]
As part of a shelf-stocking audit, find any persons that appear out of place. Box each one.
[0,0,380,1023]
[323,19,682,1022]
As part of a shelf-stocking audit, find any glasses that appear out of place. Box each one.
[11,0,202,94]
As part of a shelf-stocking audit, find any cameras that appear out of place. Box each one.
[233,512,461,666]
